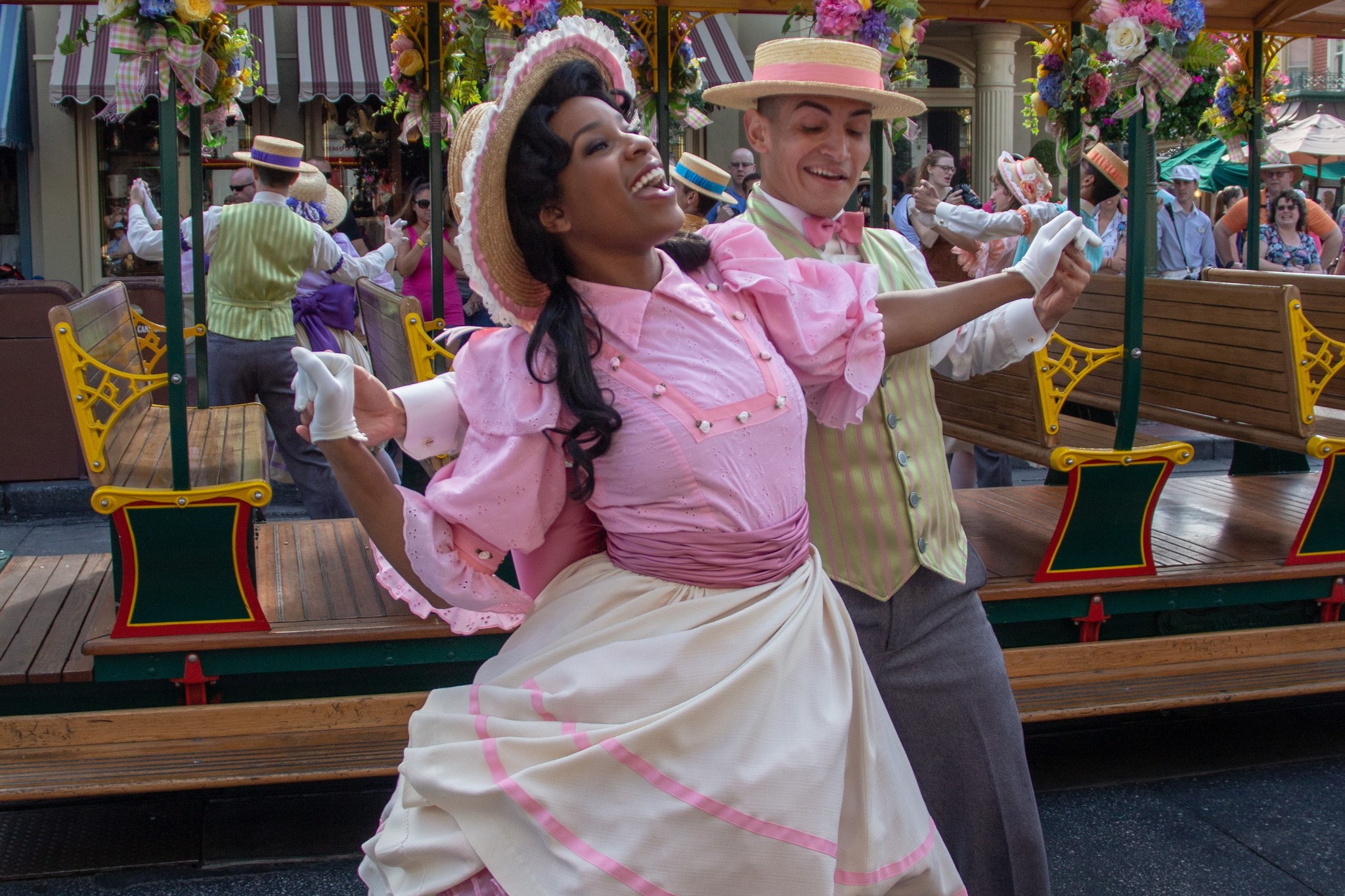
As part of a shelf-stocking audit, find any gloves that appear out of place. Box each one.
[290,346,368,445]
[1002,210,1103,299]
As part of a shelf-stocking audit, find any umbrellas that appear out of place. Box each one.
[1221,104,1345,186]
[1160,137,1345,193]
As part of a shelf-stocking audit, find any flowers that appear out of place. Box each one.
[781,0,927,148]
[57,0,264,157]
[1019,0,1293,173]
[372,0,715,149]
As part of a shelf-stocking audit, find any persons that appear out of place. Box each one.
[104,135,495,520]
[670,141,1345,489]
[295,17,1056,896]
[291,39,1093,896]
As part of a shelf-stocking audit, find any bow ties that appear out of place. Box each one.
[802,211,865,248]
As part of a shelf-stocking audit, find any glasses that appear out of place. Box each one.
[229,182,255,191]
[730,162,754,169]
[1275,205,1295,210]
[931,164,956,174]
[322,171,332,179]
[413,199,430,209]
[1267,169,1292,178]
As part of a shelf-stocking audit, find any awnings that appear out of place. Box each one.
[0,4,33,152]
[49,5,280,117]
[296,5,397,104]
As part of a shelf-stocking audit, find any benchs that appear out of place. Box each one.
[357,274,472,497]
[926,282,1194,582]
[50,277,274,635]
[1200,266,1345,477]
[1049,272,1345,565]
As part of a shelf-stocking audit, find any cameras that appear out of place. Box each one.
[951,183,983,209]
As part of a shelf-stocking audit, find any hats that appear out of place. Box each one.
[857,171,887,199]
[997,150,1053,205]
[112,222,124,230]
[701,37,927,121]
[232,135,319,173]
[286,169,348,231]
[1168,165,1200,183]
[1082,142,1129,197]
[440,16,638,328]
[1259,150,1303,185]
[669,152,738,205]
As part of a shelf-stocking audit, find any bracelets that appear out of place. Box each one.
[1283,266,1287,272]
[1225,261,1234,268]
[416,237,428,249]
